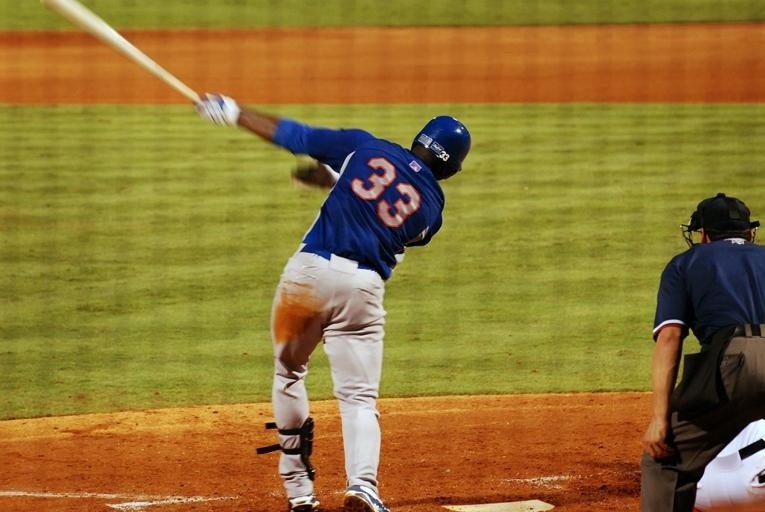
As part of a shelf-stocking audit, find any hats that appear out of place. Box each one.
[686,193,760,236]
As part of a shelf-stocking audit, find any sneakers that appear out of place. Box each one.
[286,496,321,512]
[342,485,391,512]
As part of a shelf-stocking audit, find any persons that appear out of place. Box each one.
[633,191,765,511]
[194,87,473,512]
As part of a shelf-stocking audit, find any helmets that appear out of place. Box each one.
[411,114,472,180]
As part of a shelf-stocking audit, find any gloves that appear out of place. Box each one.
[193,92,242,127]
[292,157,338,191]
